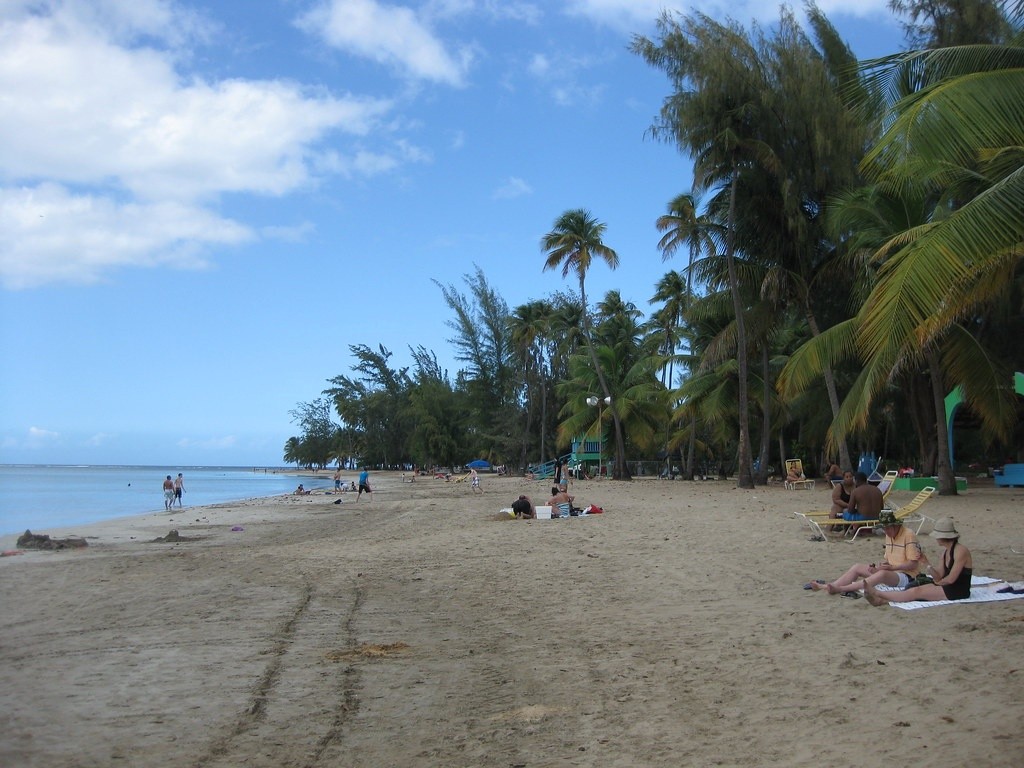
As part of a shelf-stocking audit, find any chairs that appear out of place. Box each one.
[792,469,904,533]
[808,486,937,543]
[785,460,815,491]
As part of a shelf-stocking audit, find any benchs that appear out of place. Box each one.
[992,463,1024,488]
[432,469,450,479]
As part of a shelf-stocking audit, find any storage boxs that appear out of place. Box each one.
[533,505,553,519]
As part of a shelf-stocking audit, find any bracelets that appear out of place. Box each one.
[926,565,932,569]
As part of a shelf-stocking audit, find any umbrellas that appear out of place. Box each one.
[469,460,489,472]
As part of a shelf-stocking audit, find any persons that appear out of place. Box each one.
[413,465,421,474]
[163,475,175,509]
[470,467,483,494]
[445,471,452,481]
[601,464,607,478]
[173,473,187,506]
[296,484,304,495]
[512,456,576,520]
[823,472,856,530]
[810,509,923,596]
[402,473,406,483]
[863,518,973,607]
[333,467,374,503]
[787,461,802,482]
[412,475,416,482]
[827,461,842,488]
[842,473,884,534]
